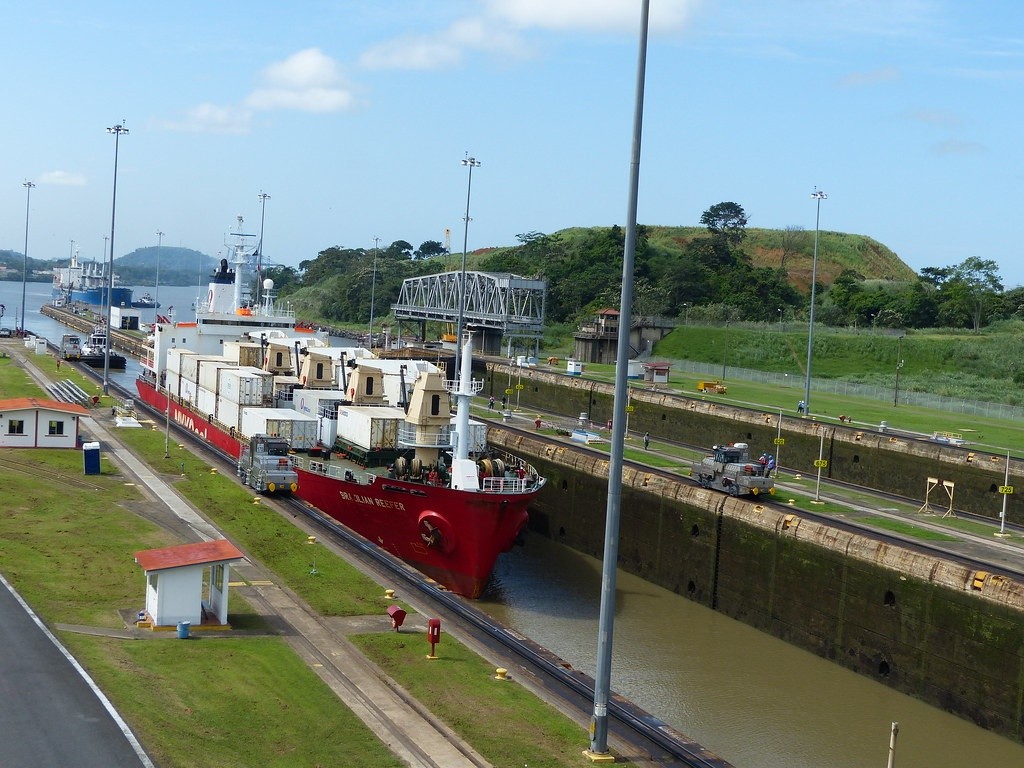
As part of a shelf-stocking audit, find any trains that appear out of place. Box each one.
[60,334,82,361]
[240,434,301,494]
[691,443,775,498]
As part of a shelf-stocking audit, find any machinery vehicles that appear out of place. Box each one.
[441,228,459,343]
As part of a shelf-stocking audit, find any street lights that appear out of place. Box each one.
[68,241,74,297]
[452,150,482,406]
[778,308,782,323]
[103,119,128,396]
[256,194,271,303]
[100,236,109,319]
[804,185,827,415]
[871,313,875,333]
[21,181,36,333]
[369,237,382,350]
[684,303,688,326]
[154,231,165,324]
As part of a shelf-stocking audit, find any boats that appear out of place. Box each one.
[131,293,160,308]
[135,216,548,599]
[81,320,119,356]
[52,251,134,308]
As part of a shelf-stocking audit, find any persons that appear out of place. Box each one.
[796,400,805,413]
[501,395,506,409]
[383,455,526,487]
[71,304,106,324]
[15,326,22,336]
[81,342,115,356]
[644,431,649,449]
[759,451,774,477]
[488,396,494,409]
